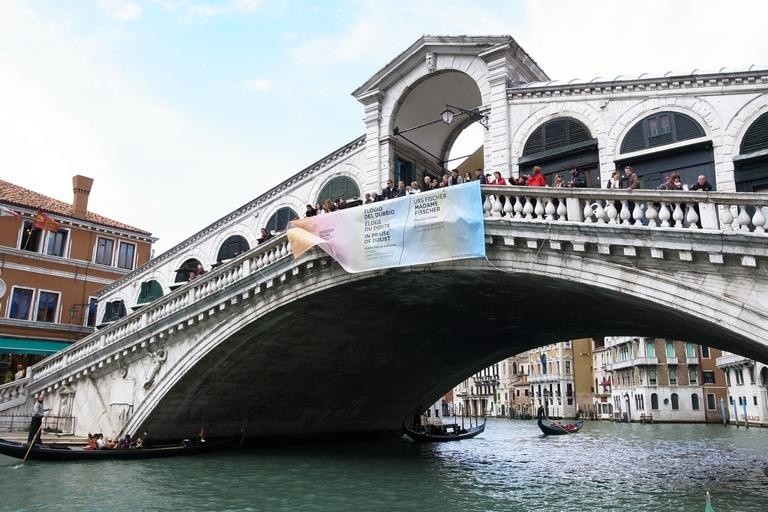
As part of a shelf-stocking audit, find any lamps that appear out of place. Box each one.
[439,104,490,130]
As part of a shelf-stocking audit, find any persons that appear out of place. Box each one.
[412,404,428,433]
[86,430,151,450]
[537,405,545,422]
[3,363,25,383]
[188,265,207,280]
[257,228,275,245]
[28,393,50,446]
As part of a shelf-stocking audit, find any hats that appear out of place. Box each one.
[452,169,458,172]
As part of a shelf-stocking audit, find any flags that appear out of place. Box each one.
[33,210,61,234]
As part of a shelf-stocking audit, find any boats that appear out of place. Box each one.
[0,430,246,462]
[537,418,584,435]
[402,418,487,442]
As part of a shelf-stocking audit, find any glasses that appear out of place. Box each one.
[387,183,390,184]
[675,176,680,178]
